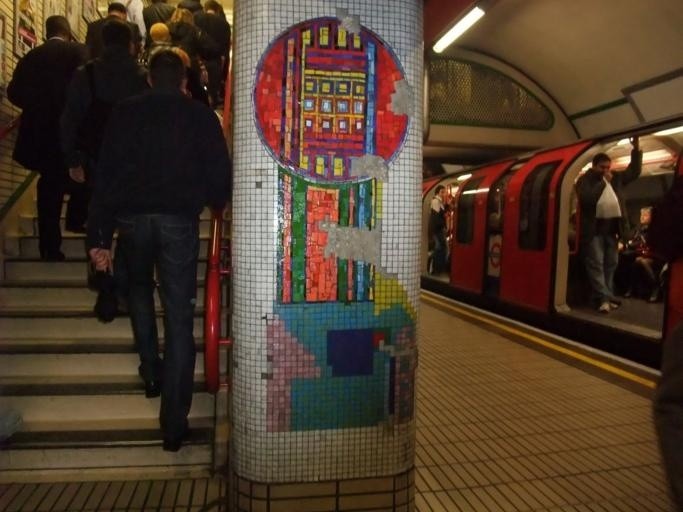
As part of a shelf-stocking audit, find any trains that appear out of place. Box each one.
[421,116,682,373]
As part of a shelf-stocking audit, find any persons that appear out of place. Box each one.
[84,2,232,323]
[570,135,671,313]
[84,45,235,452]
[428,178,458,277]
[9,16,86,262]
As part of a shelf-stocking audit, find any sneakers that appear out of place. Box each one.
[598,301,611,314]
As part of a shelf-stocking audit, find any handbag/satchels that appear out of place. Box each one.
[633,242,653,258]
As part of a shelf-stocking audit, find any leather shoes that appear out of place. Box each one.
[143,376,162,398]
[649,288,659,303]
[161,414,190,452]
